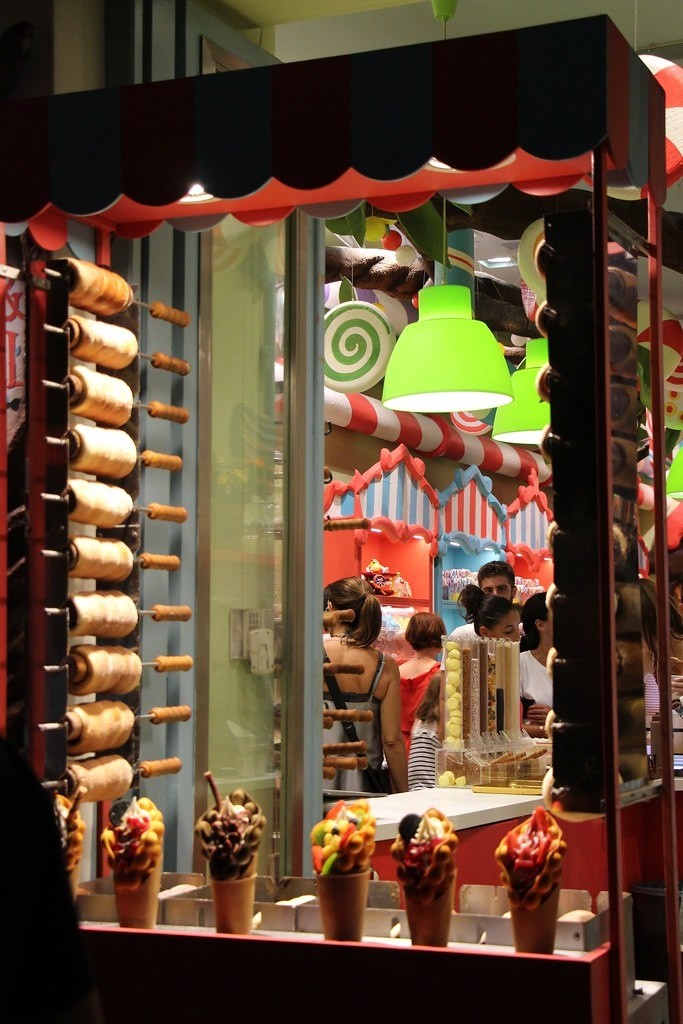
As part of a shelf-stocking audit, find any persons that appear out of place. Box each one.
[671,678,682,697]
[322,561,553,815]
[639,577,683,705]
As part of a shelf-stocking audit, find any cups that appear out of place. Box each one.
[60,872,78,901]
[511,886,561,954]
[115,860,165,929]
[405,869,456,947]
[318,869,370,941]
[213,873,258,934]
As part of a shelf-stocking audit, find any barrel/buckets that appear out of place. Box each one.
[632,881,683,983]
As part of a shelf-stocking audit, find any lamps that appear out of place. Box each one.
[666,446,683,499]
[490,338,554,443]
[379,197,514,414]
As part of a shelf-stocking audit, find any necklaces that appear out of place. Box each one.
[331,634,348,638]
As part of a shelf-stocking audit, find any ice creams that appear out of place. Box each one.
[194,770,267,934]
[494,806,569,955]
[391,809,459,949]
[100,796,166,931]
[311,798,377,942]
[53,785,88,902]
